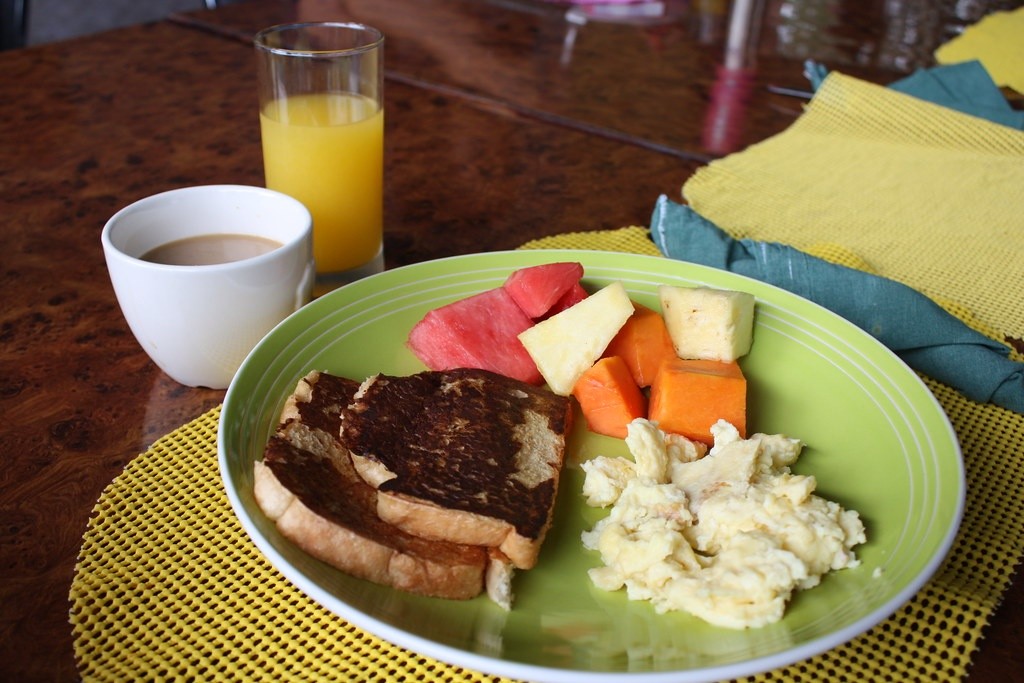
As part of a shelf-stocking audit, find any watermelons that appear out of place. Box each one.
[407,262,590,387]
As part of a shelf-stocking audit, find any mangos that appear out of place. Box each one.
[574,301,747,449]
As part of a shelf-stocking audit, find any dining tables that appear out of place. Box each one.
[0,0,1024,683]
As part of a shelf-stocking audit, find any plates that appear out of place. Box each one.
[217,249,967,683]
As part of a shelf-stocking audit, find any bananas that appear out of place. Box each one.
[517,277,755,397]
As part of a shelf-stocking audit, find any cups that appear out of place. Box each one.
[100,184,314,388]
[254,21,385,297]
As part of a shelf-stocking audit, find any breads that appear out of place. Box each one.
[257,366,575,608]
